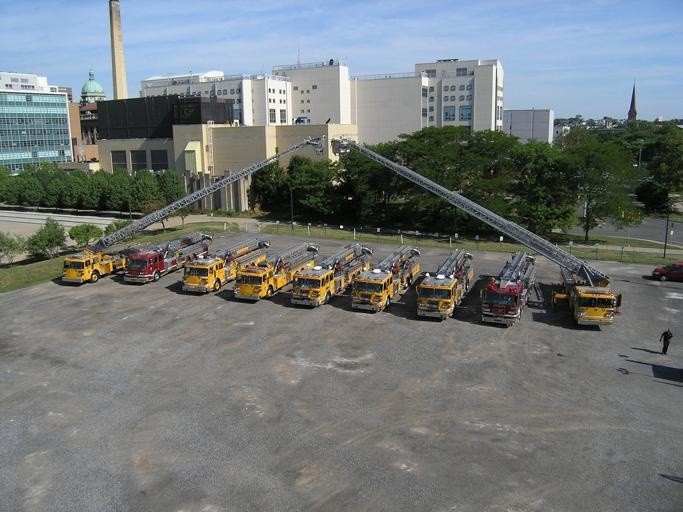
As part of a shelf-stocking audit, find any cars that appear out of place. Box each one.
[650,261,683,283]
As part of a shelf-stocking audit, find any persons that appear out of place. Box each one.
[660,328,673,355]
[516,275,522,287]
[150,258,154,266]
[391,261,400,279]
[333,260,344,276]
[275,257,285,274]
[454,267,463,282]
[225,250,233,267]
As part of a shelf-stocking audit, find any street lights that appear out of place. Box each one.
[663,222,675,258]
